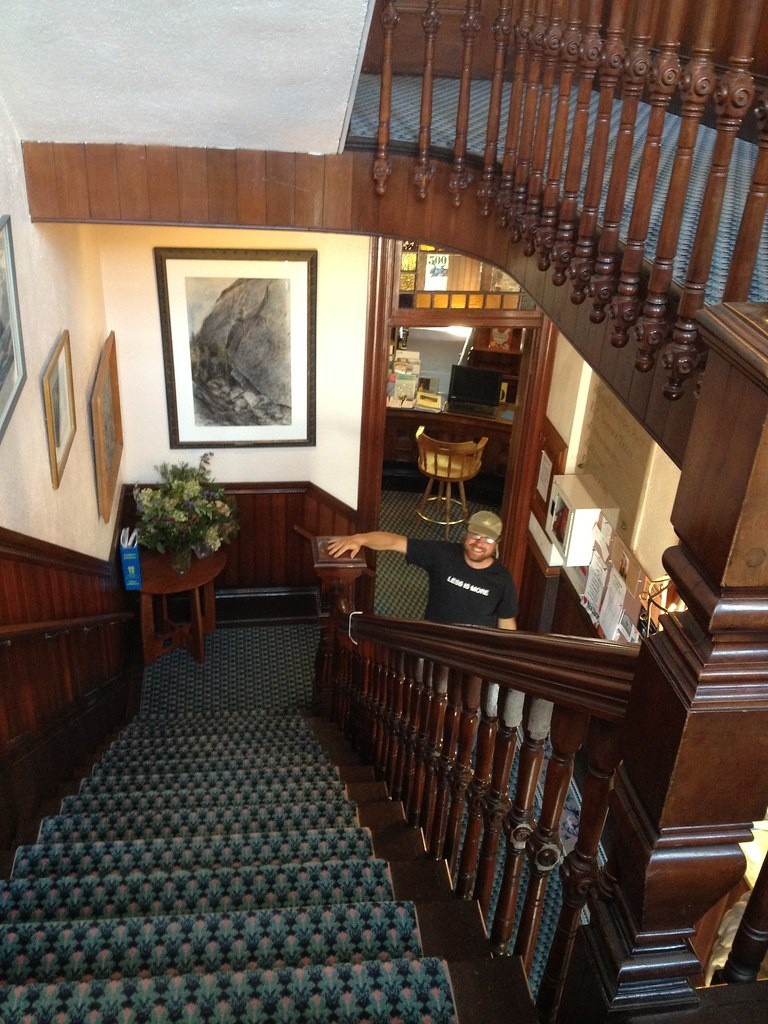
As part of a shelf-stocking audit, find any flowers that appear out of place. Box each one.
[132,453,241,560]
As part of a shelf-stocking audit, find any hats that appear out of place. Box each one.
[467,511,503,541]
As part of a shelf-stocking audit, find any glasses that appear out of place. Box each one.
[468,531,501,544]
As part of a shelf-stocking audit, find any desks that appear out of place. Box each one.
[138,547,227,666]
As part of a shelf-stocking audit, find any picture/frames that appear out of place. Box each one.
[41,329,78,491]
[0,214,28,445]
[90,329,125,527]
[152,245,320,452]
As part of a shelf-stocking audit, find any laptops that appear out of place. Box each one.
[445,364,503,418]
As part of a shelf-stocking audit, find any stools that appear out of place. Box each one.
[415,425,489,542]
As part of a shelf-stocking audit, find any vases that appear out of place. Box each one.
[172,548,192,575]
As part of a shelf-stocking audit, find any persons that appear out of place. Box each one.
[326,511,519,767]
